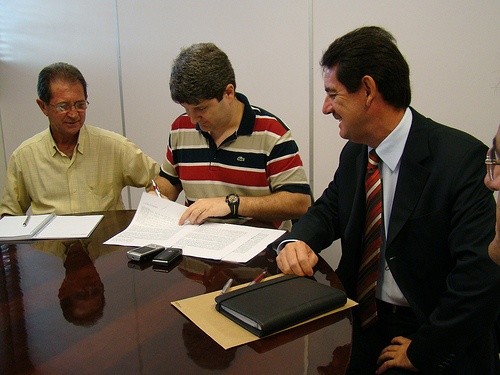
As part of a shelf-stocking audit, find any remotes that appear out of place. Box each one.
[127,243,165,261]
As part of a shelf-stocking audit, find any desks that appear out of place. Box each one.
[0,210,353,375]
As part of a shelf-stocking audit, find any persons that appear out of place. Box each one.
[0,61,158,222]
[267,26,500,375]
[178,255,282,369]
[26,210,136,326]
[149,43,314,231]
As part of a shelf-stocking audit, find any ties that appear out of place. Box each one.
[356,148,384,324]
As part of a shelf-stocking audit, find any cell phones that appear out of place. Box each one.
[152,248,182,266]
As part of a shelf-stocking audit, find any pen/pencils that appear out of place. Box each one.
[23,209,32,227]
[220,279,233,294]
[247,271,267,287]
[152,179,161,197]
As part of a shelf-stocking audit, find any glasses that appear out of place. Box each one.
[486,149,500,180]
[42,101,91,113]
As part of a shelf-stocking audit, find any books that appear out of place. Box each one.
[214,275,348,339]
[0,213,105,241]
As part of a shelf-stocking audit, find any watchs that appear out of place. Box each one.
[225,193,241,219]
